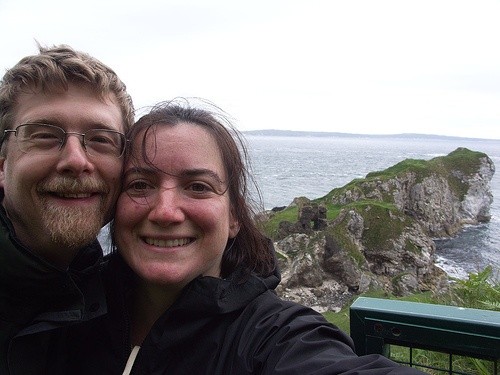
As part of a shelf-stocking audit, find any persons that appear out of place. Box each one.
[97,96,427,375]
[1,38,133,375]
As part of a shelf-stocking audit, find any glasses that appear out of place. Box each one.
[2,122,131,158]
